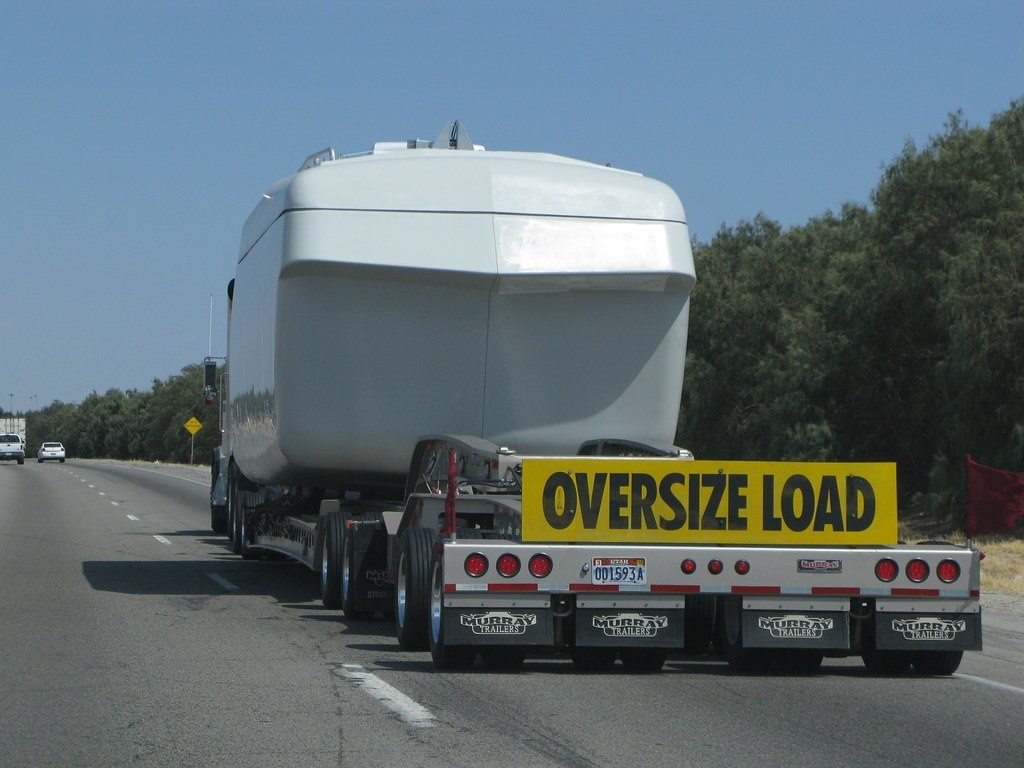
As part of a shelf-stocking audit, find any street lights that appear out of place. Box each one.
[9,393,14,418]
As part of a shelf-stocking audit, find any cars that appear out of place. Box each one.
[38,441,65,463]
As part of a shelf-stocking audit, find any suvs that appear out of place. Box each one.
[0,434,25,465]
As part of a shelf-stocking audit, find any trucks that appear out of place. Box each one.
[0,418,27,443]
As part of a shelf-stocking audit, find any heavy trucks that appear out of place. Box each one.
[200,119,986,678]
